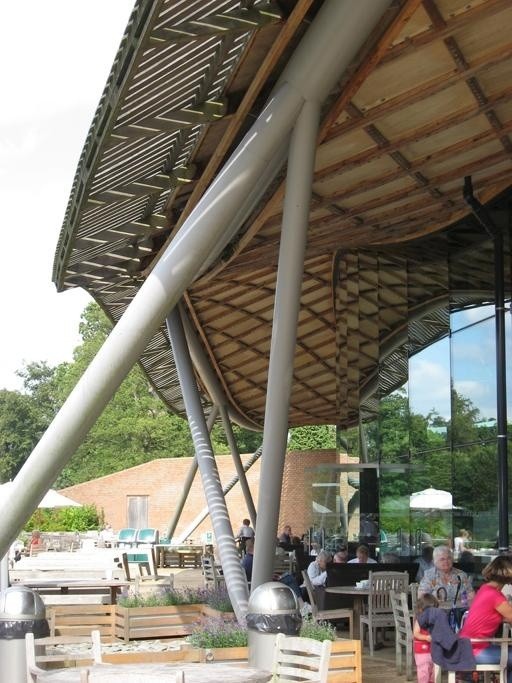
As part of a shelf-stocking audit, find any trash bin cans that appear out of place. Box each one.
[117,528,159,563]
[249,582,302,675]
[0,586,47,683]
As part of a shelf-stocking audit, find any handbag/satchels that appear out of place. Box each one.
[450,607,469,632]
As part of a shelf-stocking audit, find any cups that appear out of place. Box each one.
[289,552,295,562]
[355,579,368,589]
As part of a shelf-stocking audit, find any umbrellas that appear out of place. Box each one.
[0,477,84,510]
[381,486,464,510]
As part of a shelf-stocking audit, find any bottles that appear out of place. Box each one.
[461,590,468,609]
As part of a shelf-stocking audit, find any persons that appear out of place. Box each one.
[411,547,434,581]
[457,556,512,683]
[8,531,44,560]
[301,544,402,604]
[418,545,476,606]
[291,537,303,543]
[240,539,316,619]
[412,593,439,683]
[234,519,255,554]
[280,526,293,543]
[454,529,478,553]
